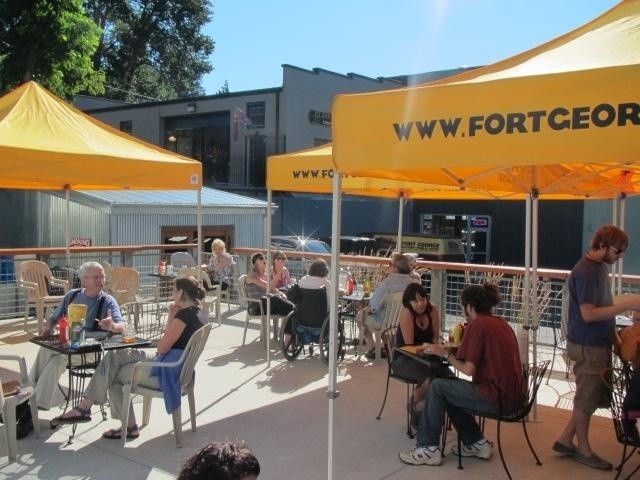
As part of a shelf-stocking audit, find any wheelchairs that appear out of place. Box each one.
[280,305,346,366]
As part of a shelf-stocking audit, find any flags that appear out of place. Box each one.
[231,107,253,142]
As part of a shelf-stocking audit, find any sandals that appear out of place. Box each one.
[52,406,92,425]
[103,425,140,439]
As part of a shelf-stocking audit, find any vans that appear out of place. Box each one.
[327,234,377,257]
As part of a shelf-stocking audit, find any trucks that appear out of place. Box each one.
[356,232,465,293]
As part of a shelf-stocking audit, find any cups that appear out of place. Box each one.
[121,324,138,343]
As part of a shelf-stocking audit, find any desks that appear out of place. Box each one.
[27,328,154,446]
[395,340,482,454]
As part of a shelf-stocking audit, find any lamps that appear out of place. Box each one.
[186,103,197,112]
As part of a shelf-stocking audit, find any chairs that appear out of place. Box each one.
[118,322,215,451]
[236,274,424,371]
[0,350,43,463]
[545,298,639,478]
[0,252,235,340]
[375,324,456,443]
[444,358,552,480]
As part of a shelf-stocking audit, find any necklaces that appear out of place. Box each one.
[415,312,426,327]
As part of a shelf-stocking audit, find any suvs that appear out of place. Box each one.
[270,234,346,280]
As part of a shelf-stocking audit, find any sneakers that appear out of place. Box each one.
[399,445,442,466]
[574,451,613,470]
[452,440,492,459]
[552,441,577,456]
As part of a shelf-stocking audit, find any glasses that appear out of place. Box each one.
[389,263,394,267]
[277,257,286,261]
[85,275,105,281]
[609,245,626,255]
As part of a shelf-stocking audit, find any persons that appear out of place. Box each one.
[0,259,127,441]
[200,235,238,291]
[396,281,528,468]
[268,249,293,295]
[49,272,207,444]
[387,282,444,432]
[244,250,296,356]
[295,257,332,315]
[401,252,421,284]
[354,252,422,361]
[168,432,268,480]
[549,223,640,473]
[621,315,640,444]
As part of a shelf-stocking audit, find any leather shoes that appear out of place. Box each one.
[365,351,375,358]
[17,420,33,439]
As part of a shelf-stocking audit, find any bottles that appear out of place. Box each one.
[158,258,199,278]
[57,312,86,345]
[338,263,386,298]
[453,321,464,344]
[278,274,302,289]
[441,340,448,346]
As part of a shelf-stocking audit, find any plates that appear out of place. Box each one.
[614,313,633,327]
